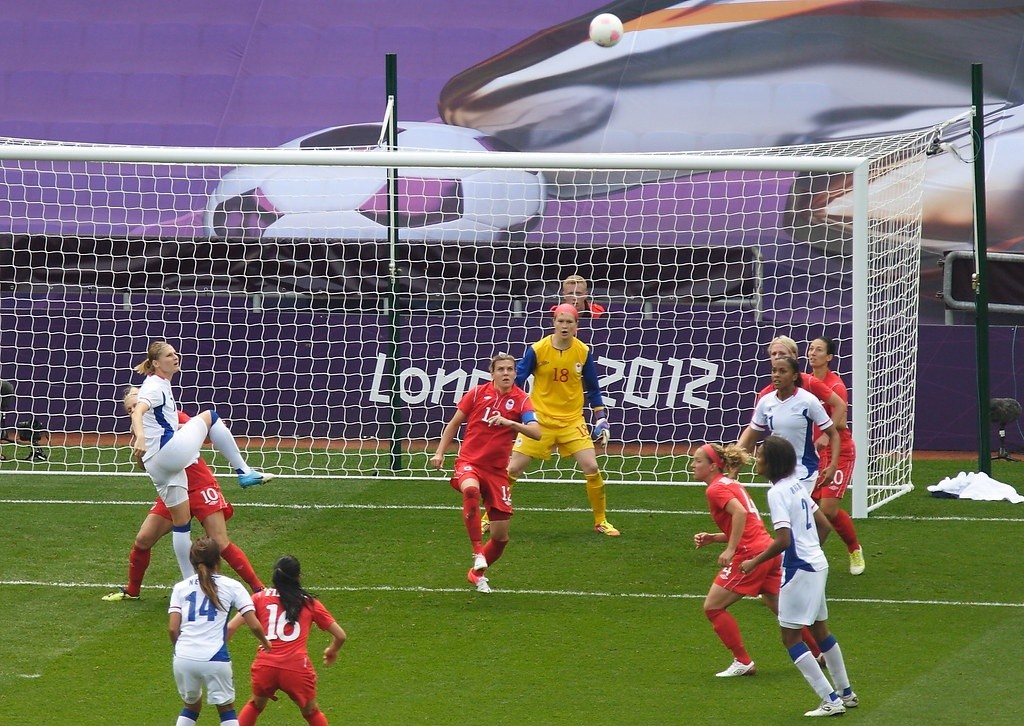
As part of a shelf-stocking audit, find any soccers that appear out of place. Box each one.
[589,13,623,47]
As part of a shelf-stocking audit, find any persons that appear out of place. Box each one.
[169,537,272,726]
[738,435,858,717]
[480,304,620,536]
[226,554,346,726]
[102,388,268,601]
[550,275,607,318]
[805,336,865,576]
[429,354,541,594]
[737,356,839,557]
[131,341,275,580]
[756,335,846,507]
[688,443,826,676]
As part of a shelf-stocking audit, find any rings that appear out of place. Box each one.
[742,571,745,574]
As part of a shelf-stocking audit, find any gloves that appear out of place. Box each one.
[593,417,610,436]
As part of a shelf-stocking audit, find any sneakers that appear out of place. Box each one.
[237,468,276,489]
[848,542,866,576]
[815,654,826,669]
[716,659,756,677]
[472,553,488,571]
[481,516,491,535]
[595,522,620,536]
[803,700,846,716]
[102,588,140,601]
[468,567,492,595]
[834,689,859,707]
